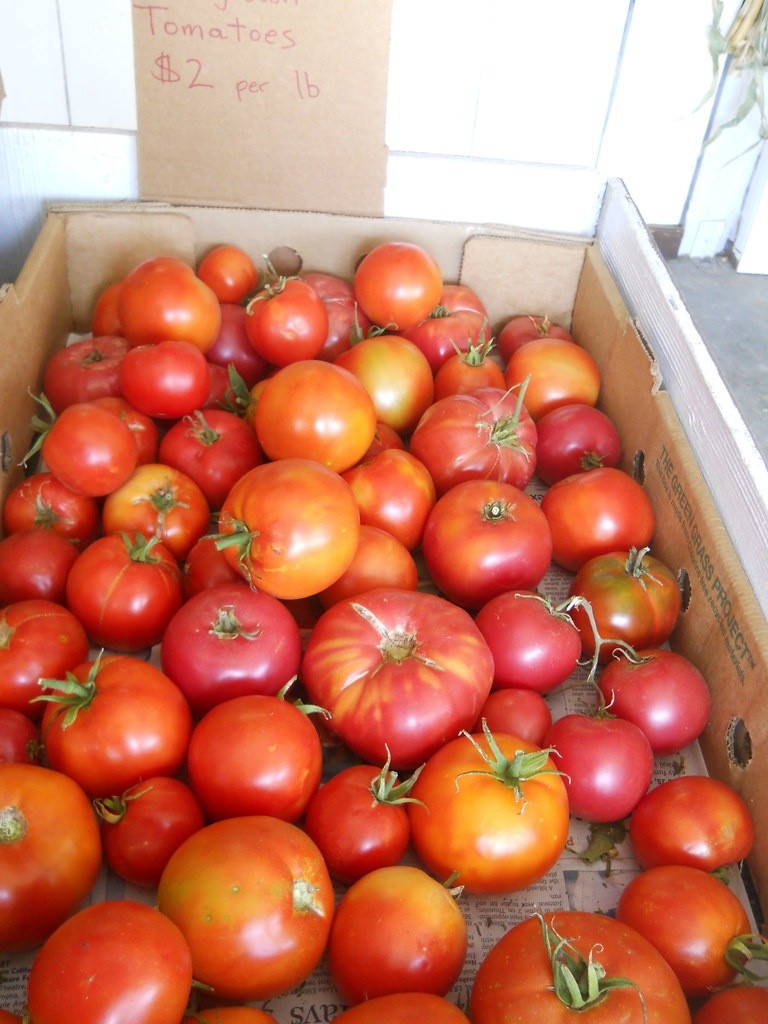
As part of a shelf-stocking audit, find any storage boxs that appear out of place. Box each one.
[0,199,768,1024]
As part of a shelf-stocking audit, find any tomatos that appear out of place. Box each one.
[1,244,767,1024]
